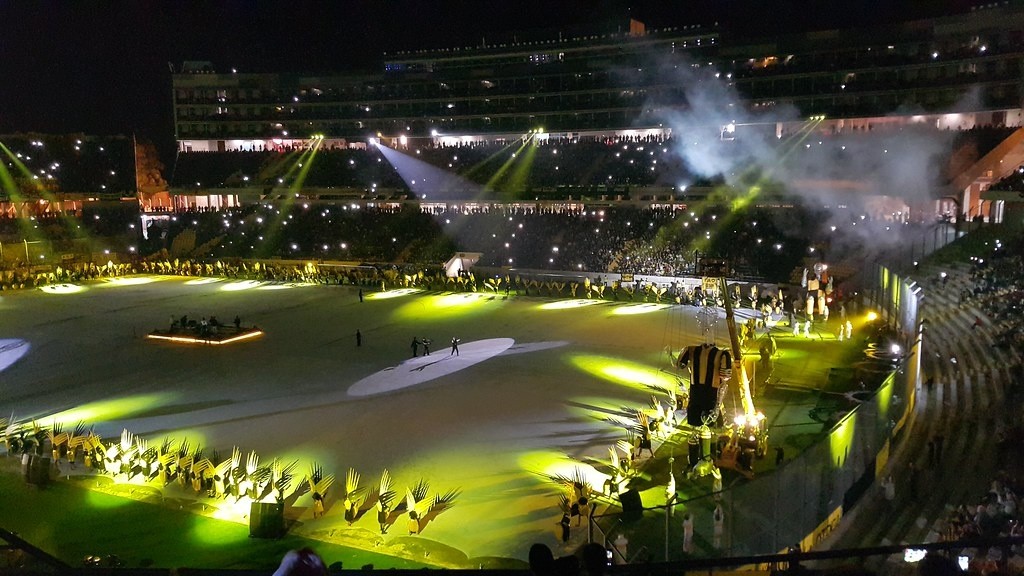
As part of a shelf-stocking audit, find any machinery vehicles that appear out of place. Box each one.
[701,261,769,480]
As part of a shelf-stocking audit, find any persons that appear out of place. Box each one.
[411,336,422,357]
[170,313,240,341]
[713,502,724,549]
[804,320,810,338]
[838,321,853,341]
[794,320,800,337]
[359,289,363,303]
[451,336,461,356]
[940,483,1024,542]
[683,511,694,554]
[712,467,724,500]
[356,328,362,347]
[423,337,430,356]
[666,468,676,518]
[839,305,846,321]
